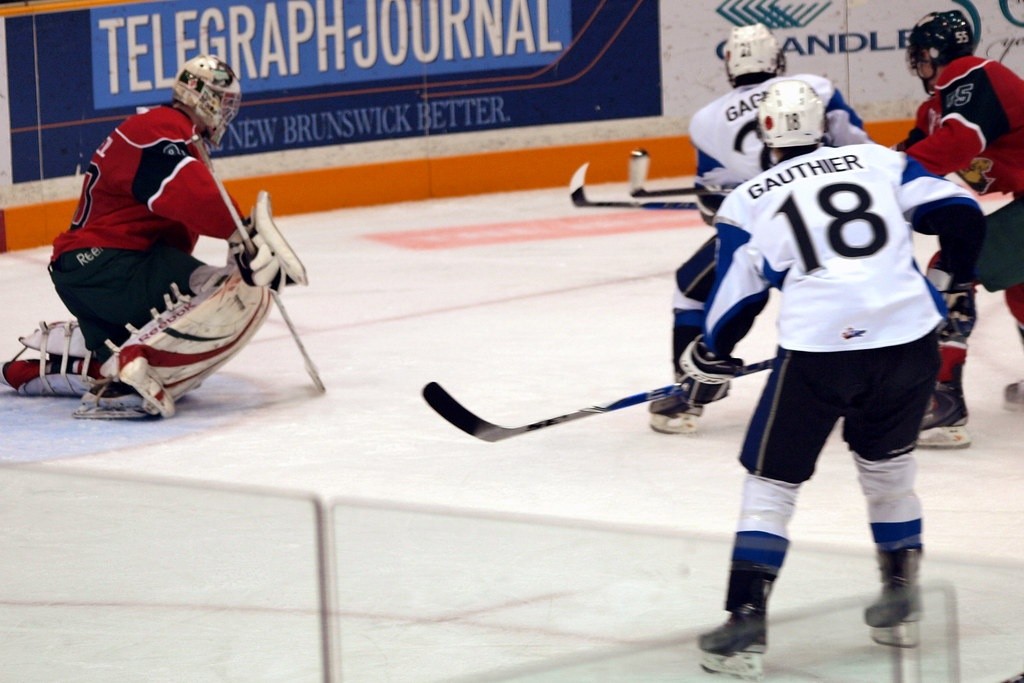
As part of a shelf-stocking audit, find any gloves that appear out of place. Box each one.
[935,270,981,342]
[668,335,744,409]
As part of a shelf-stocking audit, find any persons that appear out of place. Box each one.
[0,53,308,420]
[650,77,983,678]
[647,9,1024,451]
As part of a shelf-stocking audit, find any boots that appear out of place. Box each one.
[865,543,926,650]
[694,568,780,681]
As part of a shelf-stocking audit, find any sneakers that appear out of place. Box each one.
[73,377,153,421]
[0,352,17,390]
[645,370,704,435]
[916,362,971,450]
[1003,330,1024,413]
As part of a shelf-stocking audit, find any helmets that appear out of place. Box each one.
[718,23,786,87]
[905,8,977,69]
[752,76,830,149]
[171,53,242,146]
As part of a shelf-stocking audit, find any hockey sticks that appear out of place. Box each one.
[187,135,329,394]
[420,359,775,443]
[627,145,737,199]
[571,160,700,211]
